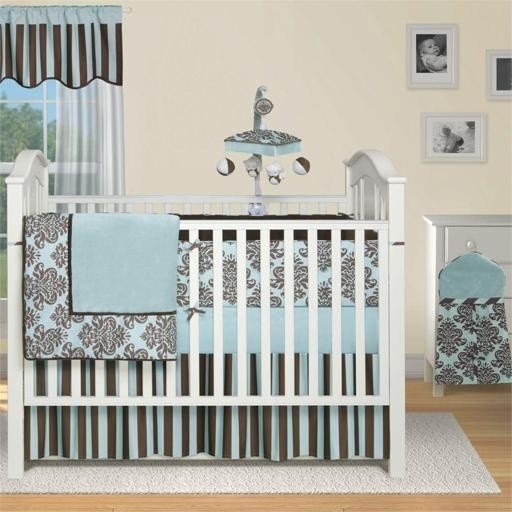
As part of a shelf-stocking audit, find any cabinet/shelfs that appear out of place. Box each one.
[421,213,512,397]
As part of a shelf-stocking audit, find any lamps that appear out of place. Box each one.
[216,83,312,216]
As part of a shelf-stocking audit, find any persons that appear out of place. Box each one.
[418,39,448,74]
[441,124,464,153]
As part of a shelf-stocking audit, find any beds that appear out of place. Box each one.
[5,148,407,481]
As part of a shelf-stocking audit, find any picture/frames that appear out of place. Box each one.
[406,23,459,90]
[483,49,512,104]
[420,111,487,162]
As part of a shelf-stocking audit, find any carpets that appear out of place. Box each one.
[0,409,501,494]
